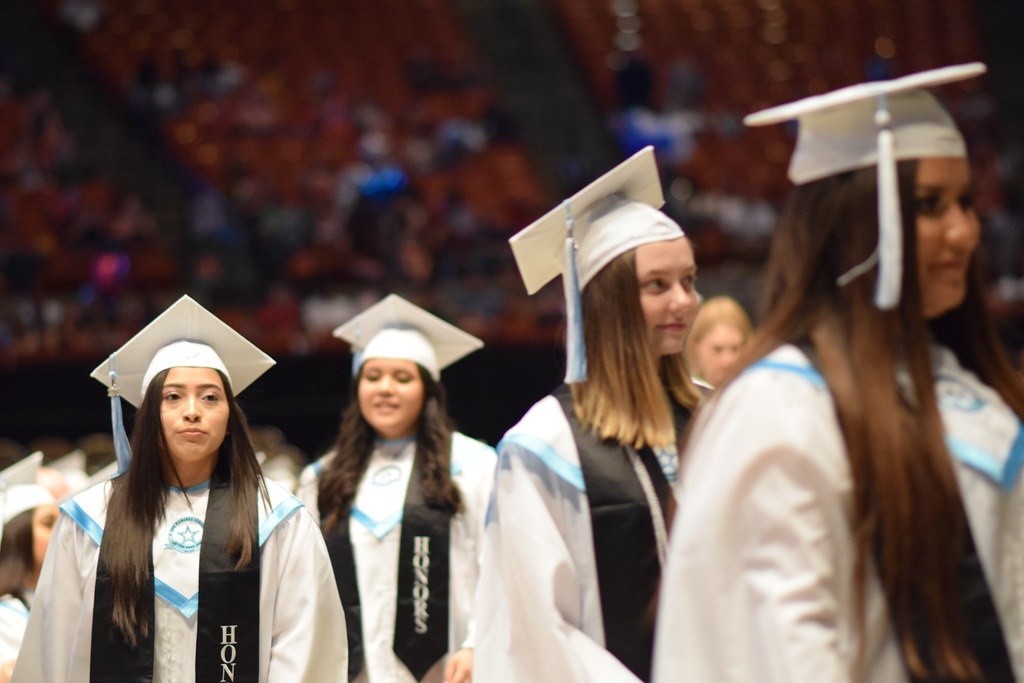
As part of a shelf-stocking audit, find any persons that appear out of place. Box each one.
[300,293,495,683]
[471,146,698,683]
[651,61,1024,683]
[0,293,348,683]
[684,296,753,392]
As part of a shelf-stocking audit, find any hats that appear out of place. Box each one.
[1,449,90,528]
[741,61,986,312]
[507,143,686,386]
[331,292,485,382]
[88,292,278,480]
